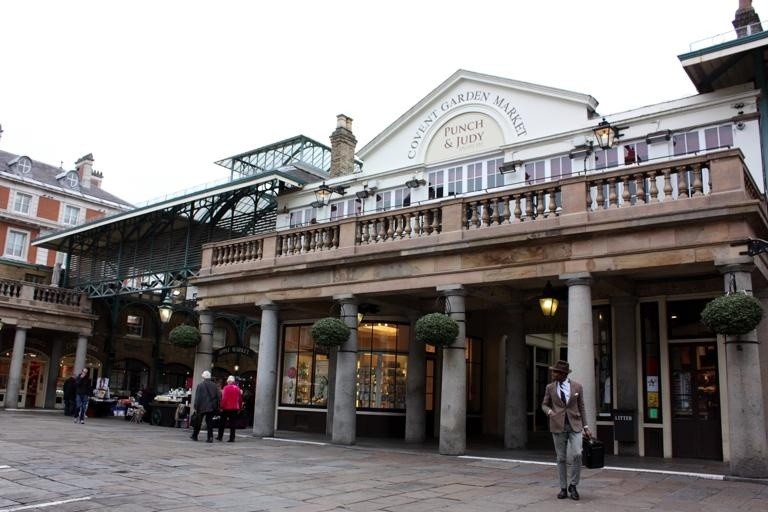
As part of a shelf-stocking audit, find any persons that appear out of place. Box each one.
[72,368,91,425]
[63,372,78,416]
[84,379,91,419]
[540,360,593,501]
[188,370,222,443]
[213,374,243,443]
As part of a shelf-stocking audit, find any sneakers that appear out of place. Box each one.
[191,434,235,444]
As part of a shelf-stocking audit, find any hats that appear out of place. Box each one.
[549,361,571,373]
[227,376,235,383]
[202,371,211,379]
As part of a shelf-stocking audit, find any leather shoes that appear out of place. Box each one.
[559,488,567,499]
[569,484,579,500]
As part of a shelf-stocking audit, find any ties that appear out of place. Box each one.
[559,382,566,405]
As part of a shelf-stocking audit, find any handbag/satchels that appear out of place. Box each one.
[112,406,126,416]
[190,410,202,427]
[582,434,604,468]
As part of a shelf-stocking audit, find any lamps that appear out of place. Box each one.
[314,182,350,206]
[157,291,199,324]
[592,118,629,151]
[537,279,561,319]
